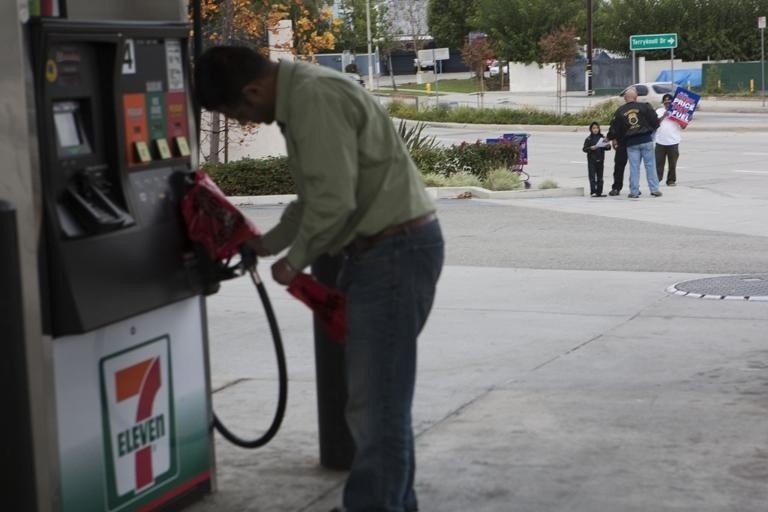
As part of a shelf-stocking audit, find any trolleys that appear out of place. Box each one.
[478,130,531,189]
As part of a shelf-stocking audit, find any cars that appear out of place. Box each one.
[488,60,509,77]
[603,81,680,111]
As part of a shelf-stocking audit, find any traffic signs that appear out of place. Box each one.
[629,33,678,51]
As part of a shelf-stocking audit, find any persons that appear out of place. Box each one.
[655,95,693,185]
[582,123,612,196]
[604,86,642,196]
[195,41,444,510]
[616,89,663,197]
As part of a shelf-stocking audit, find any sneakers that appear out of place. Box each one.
[650,191,662,196]
[666,181,676,186]
[628,190,641,198]
[591,193,601,197]
[609,189,619,196]
